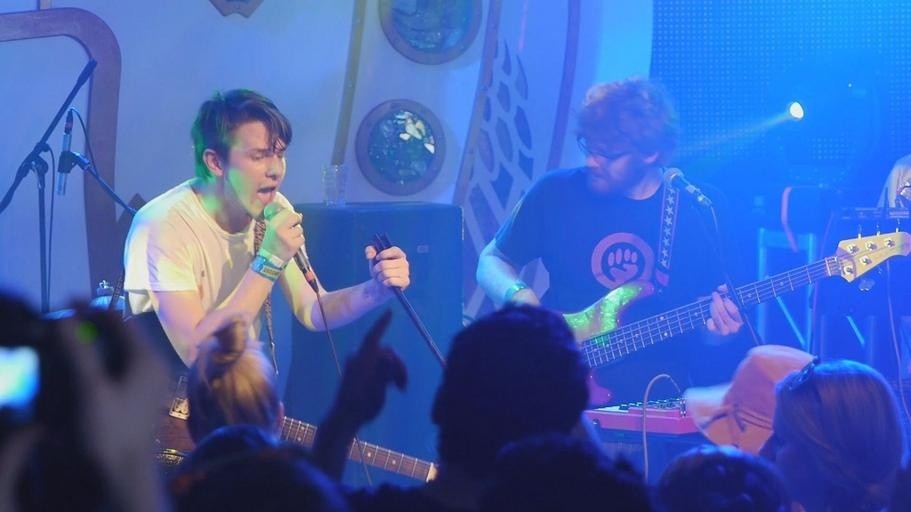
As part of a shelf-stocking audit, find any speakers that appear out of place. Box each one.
[295,202,464,488]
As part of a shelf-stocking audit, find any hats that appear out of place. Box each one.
[683,344,815,457]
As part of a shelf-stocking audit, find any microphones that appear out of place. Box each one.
[57,107,74,194]
[263,203,320,290]
[660,168,713,208]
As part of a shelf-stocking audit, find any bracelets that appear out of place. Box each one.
[249,247,288,284]
[500,277,533,305]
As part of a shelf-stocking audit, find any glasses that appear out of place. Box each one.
[575,134,639,161]
[787,356,829,434]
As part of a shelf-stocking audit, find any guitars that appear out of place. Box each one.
[562,219,910,405]
[122,310,438,482]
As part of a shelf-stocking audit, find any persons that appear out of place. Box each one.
[1,283,910,512]
[124,86,410,371]
[476,75,746,413]
[876,150,911,210]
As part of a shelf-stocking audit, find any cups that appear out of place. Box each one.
[320,163,349,209]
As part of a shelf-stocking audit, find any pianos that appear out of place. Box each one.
[584,398,700,440]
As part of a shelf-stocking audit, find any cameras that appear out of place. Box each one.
[0,285,122,439]
